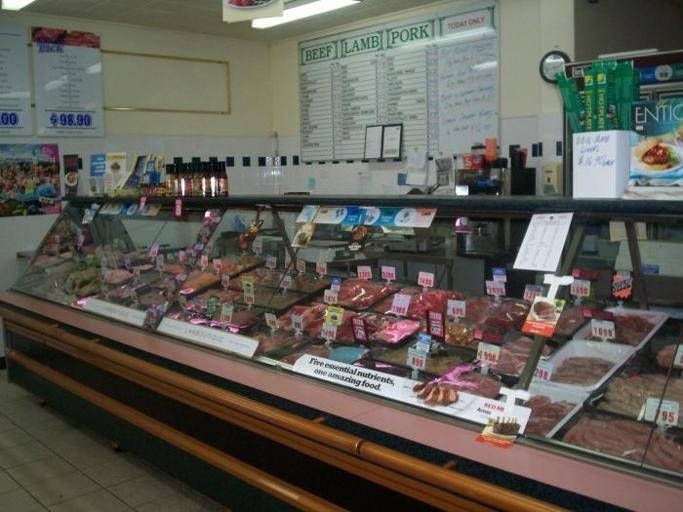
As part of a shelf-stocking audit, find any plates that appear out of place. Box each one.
[630,143,683,174]
[64,172,78,187]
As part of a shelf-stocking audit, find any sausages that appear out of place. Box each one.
[526,356,682,472]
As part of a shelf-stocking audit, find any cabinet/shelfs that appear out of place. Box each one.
[2,194,682,512]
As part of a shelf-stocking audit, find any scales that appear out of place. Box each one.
[295,239,368,263]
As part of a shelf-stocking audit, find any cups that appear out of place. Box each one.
[463,154,472,168]
[511,150,524,168]
[485,137,497,168]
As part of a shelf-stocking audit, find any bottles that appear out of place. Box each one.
[166,162,229,198]
[138,183,165,197]
[471,145,486,168]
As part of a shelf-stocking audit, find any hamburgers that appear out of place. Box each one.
[635,138,673,170]
